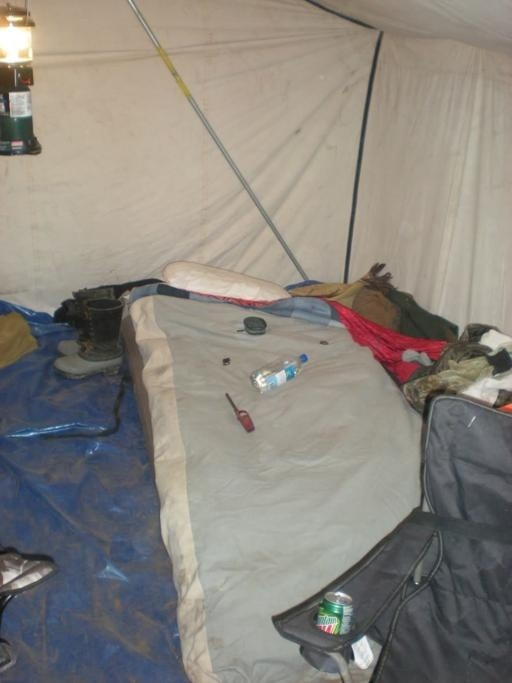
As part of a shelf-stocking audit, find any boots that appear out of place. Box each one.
[58,288,114,356]
[53,298,123,379]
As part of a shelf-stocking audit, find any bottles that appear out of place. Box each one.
[251,352,308,392]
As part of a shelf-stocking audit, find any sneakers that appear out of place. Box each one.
[1,552,56,598]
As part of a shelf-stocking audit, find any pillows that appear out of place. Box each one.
[164,259,289,306]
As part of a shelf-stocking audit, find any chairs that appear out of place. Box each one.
[272,392,512,683]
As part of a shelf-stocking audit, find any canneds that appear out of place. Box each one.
[314,590,354,635]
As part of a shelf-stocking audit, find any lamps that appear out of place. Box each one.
[0,0,42,156]
